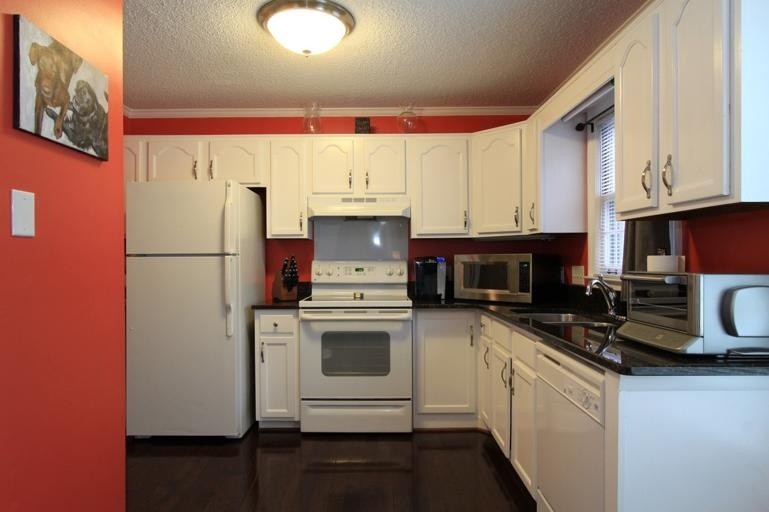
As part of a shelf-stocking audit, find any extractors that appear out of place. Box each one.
[307,194,411,221]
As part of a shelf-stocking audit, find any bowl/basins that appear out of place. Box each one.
[646,255,679,273]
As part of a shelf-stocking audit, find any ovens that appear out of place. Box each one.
[299,309,413,401]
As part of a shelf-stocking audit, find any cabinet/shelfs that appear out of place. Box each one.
[254,310,300,429]
[478,312,495,431]
[313,138,354,194]
[208,139,262,185]
[614,12,660,214]
[363,137,405,194]
[495,319,511,461]
[511,329,536,500]
[269,137,304,235]
[661,0,731,204]
[149,139,202,181]
[525,121,538,231]
[414,137,470,235]
[417,312,476,414]
[125,142,142,182]
[538,341,768,512]
[477,129,520,234]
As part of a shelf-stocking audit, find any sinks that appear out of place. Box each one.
[522,312,593,325]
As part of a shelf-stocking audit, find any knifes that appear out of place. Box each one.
[272,256,299,303]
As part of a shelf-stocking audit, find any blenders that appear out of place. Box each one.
[126,180,265,445]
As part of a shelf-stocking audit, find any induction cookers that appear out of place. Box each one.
[299,292,413,307]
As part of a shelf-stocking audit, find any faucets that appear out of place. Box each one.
[584,277,613,312]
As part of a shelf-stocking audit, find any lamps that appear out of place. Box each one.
[255,0,355,56]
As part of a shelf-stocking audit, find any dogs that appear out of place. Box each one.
[29,37,83,139]
[46,80,109,158]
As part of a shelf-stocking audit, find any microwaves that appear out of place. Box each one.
[454,253,564,307]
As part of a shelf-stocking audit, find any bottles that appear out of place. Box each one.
[437,258,446,299]
